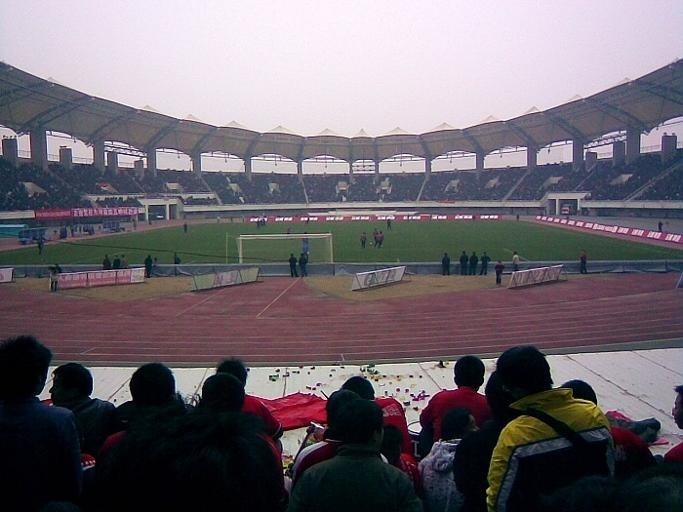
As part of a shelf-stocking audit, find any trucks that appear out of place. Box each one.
[139,209,165,221]
[561,204,575,215]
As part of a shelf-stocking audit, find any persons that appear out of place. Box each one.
[657,221,663,232]
[102,254,111,270]
[49,270,57,291]
[255,218,259,229]
[144,255,152,278]
[120,254,128,268]
[152,257,157,268]
[112,254,120,269]
[359,231,367,248]
[494,260,504,286]
[174,255,181,264]
[288,253,298,277]
[133,219,137,232]
[298,253,307,278]
[372,227,379,237]
[379,231,384,247]
[286,223,294,241]
[579,248,587,274]
[478,251,491,274]
[302,231,309,255]
[469,251,478,275]
[28,225,125,256]
[441,253,450,275]
[373,232,380,247]
[459,250,468,275]
[386,219,392,231]
[55,263,61,273]
[512,251,518,271]
[182,221,187,232]
